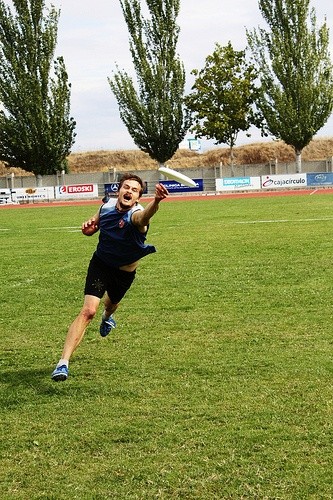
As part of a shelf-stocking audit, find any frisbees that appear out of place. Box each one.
[160,168,198,189]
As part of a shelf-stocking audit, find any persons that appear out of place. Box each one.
[51,174,169,381]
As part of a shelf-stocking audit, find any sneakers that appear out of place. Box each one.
[100,311,115,337]
[52,365,68,382]
